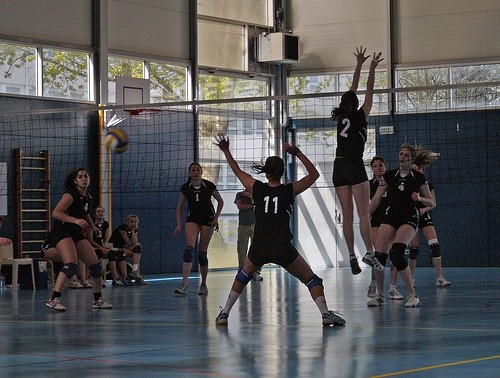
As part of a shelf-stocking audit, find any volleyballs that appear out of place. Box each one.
[105,129,129,154]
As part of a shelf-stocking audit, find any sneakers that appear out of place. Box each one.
[405,296,421,307]
[198,285,208,295]
[93,298,113,308]
[68,282,84,288]
[216,307,228,324]
[362,253,385,271]
[82,281,93,288]
[349,255,362,275]
[174,285,188,294]
[103,284,106,288]
[46,298,66,310]
[322,310,346,325]
[113,280,124,286]
[367,284,377,298]
[389,290,403,299]
[253,271,263,281]
[368,295,387,306]
[436,278,451,287]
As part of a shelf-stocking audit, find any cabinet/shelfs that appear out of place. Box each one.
[15,147,52,259]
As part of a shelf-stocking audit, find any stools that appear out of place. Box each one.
[0,256,37,291]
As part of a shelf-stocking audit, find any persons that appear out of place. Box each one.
[86,206,134,287]
[172,162,224,297]
[40,232,92,289]
[366,142,436,307]
[407,145,451,288]
[212,131,346,326]
[367,155,404,299]
[329,44,385,274]
[46,167,113,311]
[233,187,264,280]
[108,215,148,286]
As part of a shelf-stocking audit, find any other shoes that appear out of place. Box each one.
[135,277,148,285]
[124,277,133,286]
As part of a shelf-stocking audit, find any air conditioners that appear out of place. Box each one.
[256,32,300,64]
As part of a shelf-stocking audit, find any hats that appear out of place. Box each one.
[266,157,284,171]
